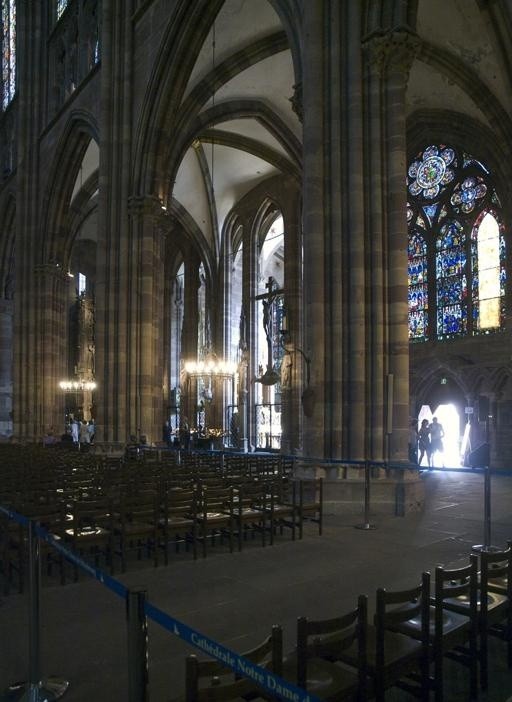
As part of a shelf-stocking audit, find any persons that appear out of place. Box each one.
[428,417,446,469]
[228,409,241,449]
[41,407,195,451]
[262,292,276,337]
[417,418,431,468]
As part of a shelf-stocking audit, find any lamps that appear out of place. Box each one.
[59,382,96,394]
[185,20,238,380]
